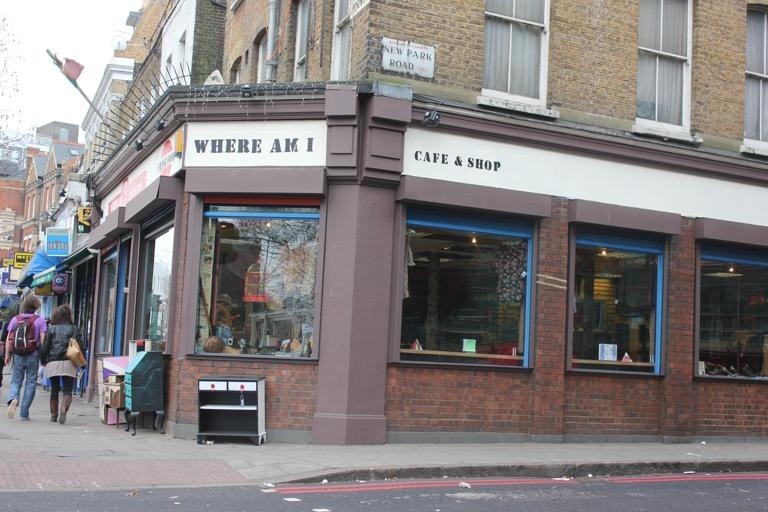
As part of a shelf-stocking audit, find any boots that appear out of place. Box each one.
[59,397,72,424]
[50,398,59,424]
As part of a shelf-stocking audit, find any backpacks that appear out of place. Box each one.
[8,314,40,354]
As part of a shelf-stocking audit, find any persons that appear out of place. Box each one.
[3,295,47,421]
[0,312,9,388]
[40,304,86,424]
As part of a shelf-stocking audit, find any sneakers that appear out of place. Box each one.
[20,417,30,421]
[7,399,18,419]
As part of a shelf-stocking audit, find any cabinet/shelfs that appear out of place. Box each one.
[197,377,267,446]
[124,352,165,435]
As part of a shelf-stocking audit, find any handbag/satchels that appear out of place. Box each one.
[65,325,86,368]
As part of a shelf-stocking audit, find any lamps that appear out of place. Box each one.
[133,139,143,151]
[157,119,167,131]
[58,188,66,197]
[423,111,440,128]
[241,85,254,98]
